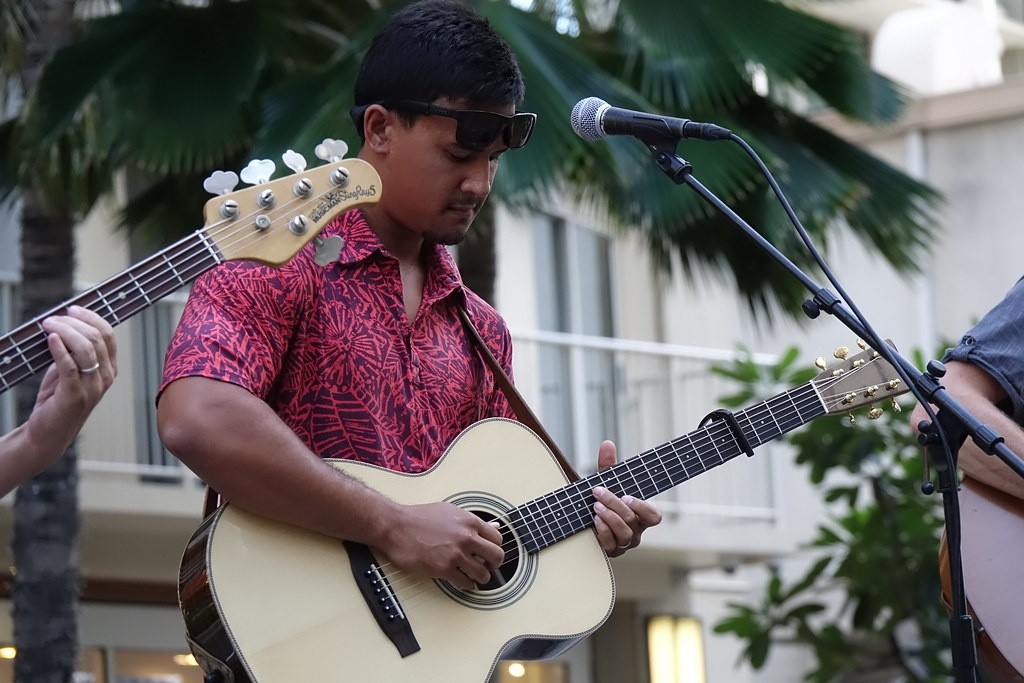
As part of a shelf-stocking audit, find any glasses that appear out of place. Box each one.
[394,100,537,149]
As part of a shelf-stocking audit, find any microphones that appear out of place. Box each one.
[571,96,732,142]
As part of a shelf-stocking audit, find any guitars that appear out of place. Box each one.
[175,336,917,682]
[937,471,1024,683]
[0,136,384,398]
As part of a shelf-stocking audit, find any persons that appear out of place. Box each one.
[910,268,1024,501]
[0,304,118,499]
[156,4,662,594]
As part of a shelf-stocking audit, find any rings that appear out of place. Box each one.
[617,541,633,549]
[80,363,100,374]
